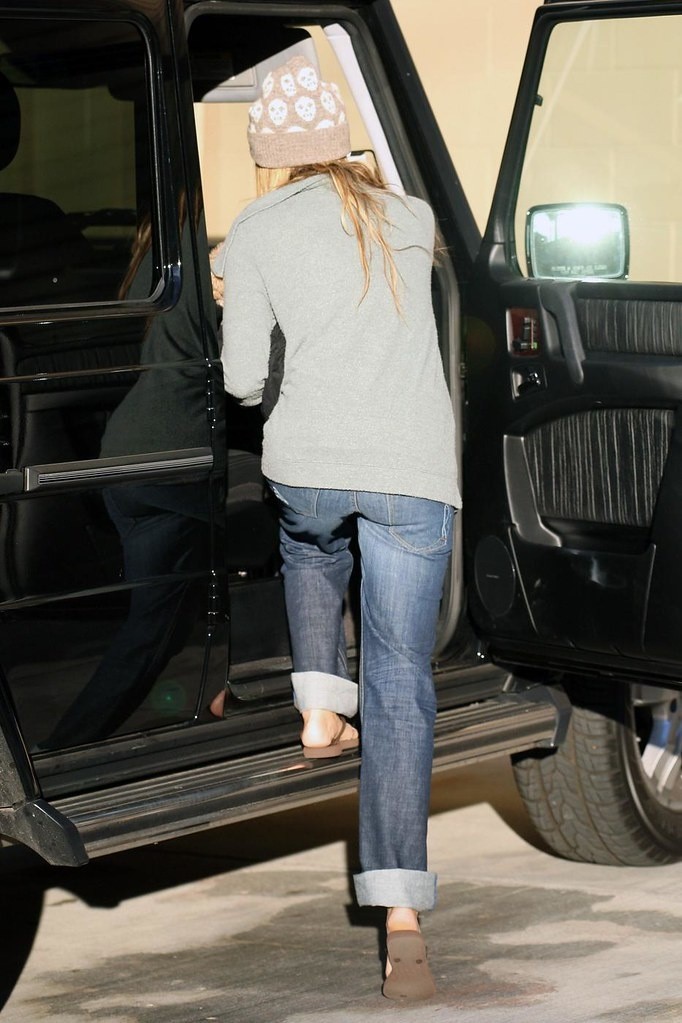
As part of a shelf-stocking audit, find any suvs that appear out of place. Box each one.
[0,1,682,868]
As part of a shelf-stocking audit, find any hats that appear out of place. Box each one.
[249,52,357,168]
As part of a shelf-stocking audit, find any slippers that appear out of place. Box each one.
[380,908,437,1003]
[300,716,361,759]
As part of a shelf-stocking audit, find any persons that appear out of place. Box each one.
[220,51,461,1002]
[35,181,225,753]
